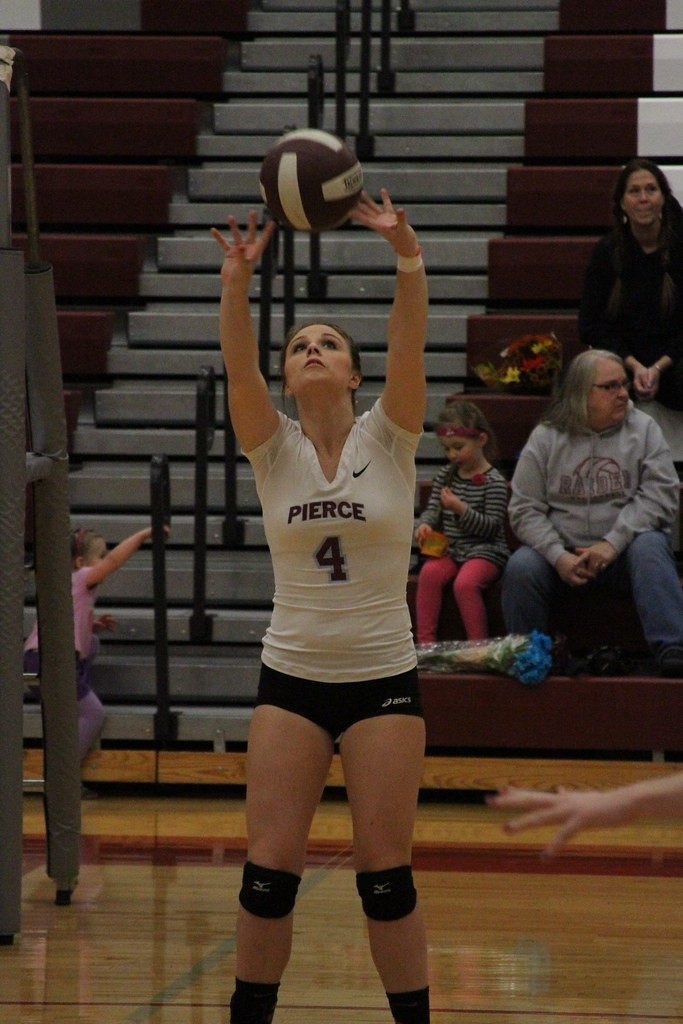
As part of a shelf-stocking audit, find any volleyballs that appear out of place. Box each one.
[258,128,363,234]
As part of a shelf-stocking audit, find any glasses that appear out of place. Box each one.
[591,378,633,395]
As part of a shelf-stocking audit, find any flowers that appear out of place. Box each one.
[413,630,556,685]
[475,333,557,395]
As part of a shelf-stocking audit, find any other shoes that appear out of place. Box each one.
[656,643,683,675]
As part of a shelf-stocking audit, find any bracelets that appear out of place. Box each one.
[396,246,423,273]
[654,364,663,371]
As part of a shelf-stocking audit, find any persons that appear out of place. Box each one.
[20,525,171,801]
[499,349,683,677]
[412,399,512,647]
[208,186,432,1024]
[578,155,683,478]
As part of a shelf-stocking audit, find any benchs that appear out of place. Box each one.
[0,0,683,761]
